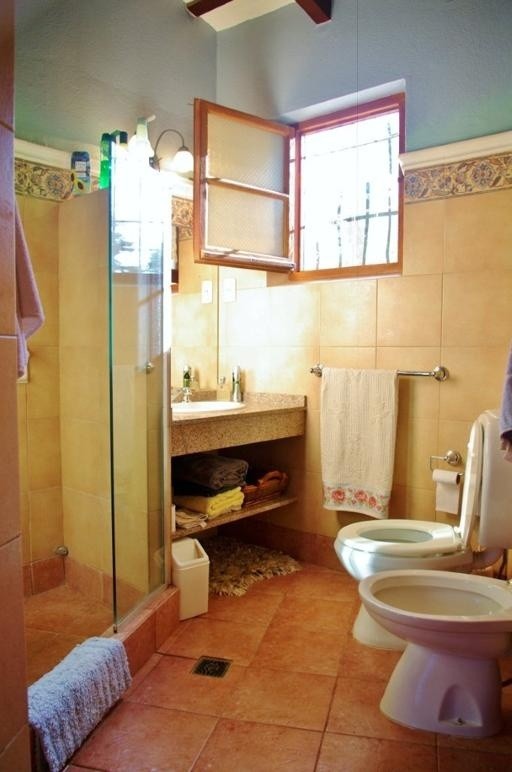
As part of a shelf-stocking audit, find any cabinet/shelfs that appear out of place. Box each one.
[232,365,241,401]
[183,365,191,388]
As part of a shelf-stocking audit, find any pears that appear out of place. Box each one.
[98,132,110,190]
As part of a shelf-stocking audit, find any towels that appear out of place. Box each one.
[170,538,211,622]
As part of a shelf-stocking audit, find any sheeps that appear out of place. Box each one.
[172,387,192,403]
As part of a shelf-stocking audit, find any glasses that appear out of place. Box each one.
[149,128,193,173]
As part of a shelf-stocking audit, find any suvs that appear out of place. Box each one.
[128,118,155,168]
